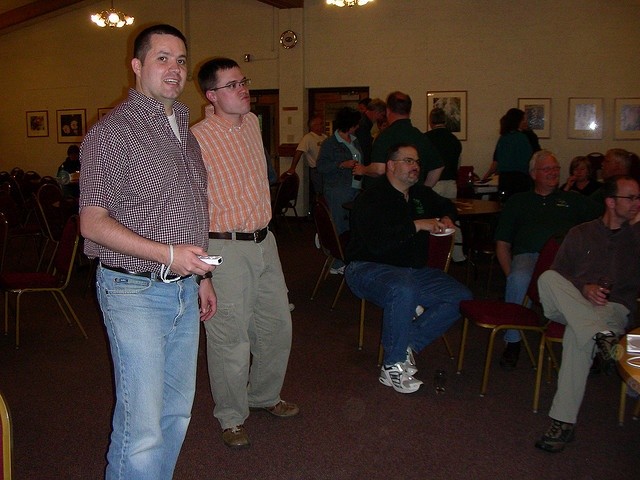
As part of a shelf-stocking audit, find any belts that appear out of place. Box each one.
[207,227,275,243]
[99,260,200,284]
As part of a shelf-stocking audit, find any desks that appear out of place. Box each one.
[616,327,640,421]
[450,196,502,215]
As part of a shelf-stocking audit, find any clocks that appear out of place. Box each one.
[279,30,297,49]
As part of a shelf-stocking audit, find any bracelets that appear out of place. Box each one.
[199,271,212,280]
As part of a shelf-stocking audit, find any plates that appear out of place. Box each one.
[429,228,455,237]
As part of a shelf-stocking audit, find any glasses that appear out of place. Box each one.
[206,77,254,93]
[388,158,422,166]
[532,165,562,172]
[607,194,640,201]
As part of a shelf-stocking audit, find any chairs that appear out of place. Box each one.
[310,201,347,310]
[458,238,560,414]
[533,322,625,427]
[3,216,89,349]
[0,389,13,479]
[32,191,58,274]
[38,184,62,205]
[358,226,455,367]
[41,175,61,189]
[0,166,31,223]
[467,232,496,290]
[272,174,300,225]
[27,170,41,188]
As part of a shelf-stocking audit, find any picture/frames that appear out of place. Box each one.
[613,97,640,140]
[26,110,49,137]
[568,96,603,141]
[98,107,115,120]
[518,98,552,139]
[56,109,87,144]
[427,90,467,141]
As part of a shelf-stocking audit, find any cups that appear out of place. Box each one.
[352,153,359,162]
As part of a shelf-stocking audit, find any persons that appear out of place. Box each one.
[352,91,424,190]
[479,114,534,196]
[506,108,540,155]
[77,23,218,480]
[559,156,601,223]
[424,108,466,266]
[344,144,473,394]
[287,114,329,249]
[355,98,386,155]
[357,98,372,115]
[495,149,582,370]
[189,58,300,451]
[593,148,638,219]
[58,145,80,175]
[534,174,639,453]
[316,106,365,275]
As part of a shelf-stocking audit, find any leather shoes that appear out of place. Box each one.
[251,399,300,418]
[222,425,253,450]
[500,342,520,371]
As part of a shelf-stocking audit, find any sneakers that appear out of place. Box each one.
[403,348,419,376]
[594,330,618,375]
[534,421,576,453]
[378,362,424,395]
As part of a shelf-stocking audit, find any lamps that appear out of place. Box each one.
[323,0,376,9]
[90,0,135,29]
